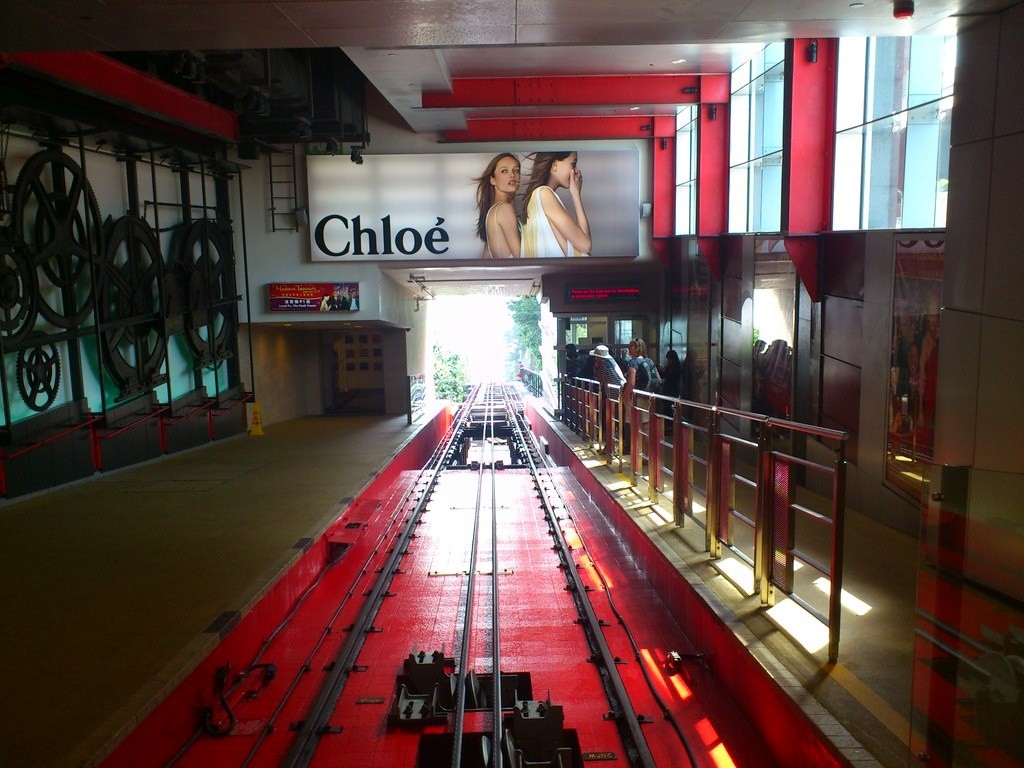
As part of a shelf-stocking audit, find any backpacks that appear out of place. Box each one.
[633,357,659,399]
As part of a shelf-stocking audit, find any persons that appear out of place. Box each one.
[684,351,705,423]
[320,290,359,311]
[752,340,793,435]
[471,151,592,258]
[566,338,683,465]
[517,362,523,378]
[887,279,940,466]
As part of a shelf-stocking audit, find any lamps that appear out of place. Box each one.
[326,138,339,155]
[351,145,365,164]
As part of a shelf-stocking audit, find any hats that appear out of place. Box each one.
[589,345,614,360]
